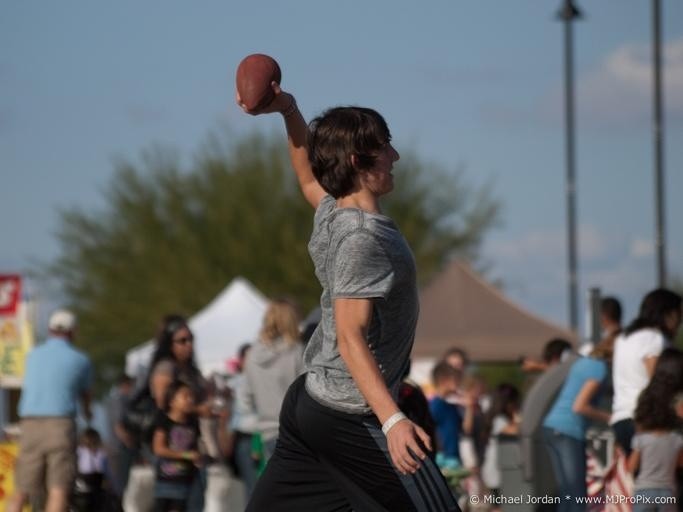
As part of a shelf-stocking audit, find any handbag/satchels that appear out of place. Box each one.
[119,398,165,440]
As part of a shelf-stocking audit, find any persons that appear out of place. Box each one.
[8,309,102,512]
[235,81,463,512]
[431,288,682,512]
[80,297,316,512]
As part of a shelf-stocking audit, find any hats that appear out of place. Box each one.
[48,309,75,333]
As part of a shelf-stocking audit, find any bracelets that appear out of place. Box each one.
[382,413,405,435]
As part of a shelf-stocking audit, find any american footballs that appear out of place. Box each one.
[236,54,281,112]
